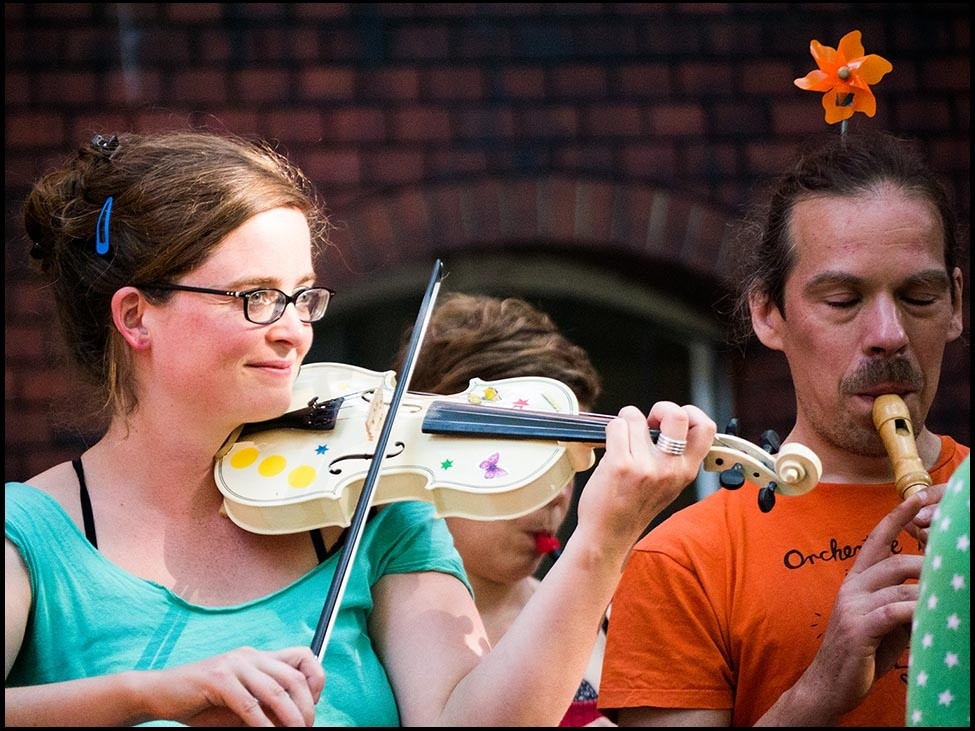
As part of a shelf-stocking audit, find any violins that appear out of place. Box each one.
[212,361,824,538]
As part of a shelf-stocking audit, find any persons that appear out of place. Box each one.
[598,129,974,727]
[395,284,617,726]
[0,120,718,727]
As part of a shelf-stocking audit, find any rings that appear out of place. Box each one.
[655,434,689,455]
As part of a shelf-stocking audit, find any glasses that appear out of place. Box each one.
[148,281,336,325]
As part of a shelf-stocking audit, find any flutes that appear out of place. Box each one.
[875,391,934,548]
